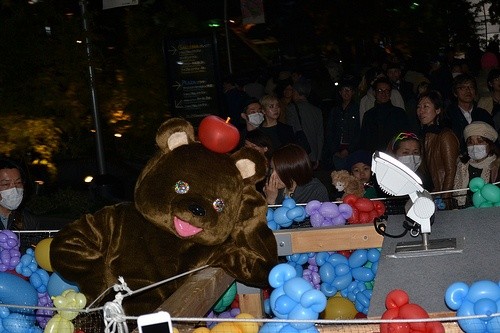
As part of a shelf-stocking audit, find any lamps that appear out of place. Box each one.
[371,151,458,254]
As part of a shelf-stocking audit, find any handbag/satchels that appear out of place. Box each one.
[294,131,311,155]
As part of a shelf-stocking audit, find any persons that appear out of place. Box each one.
[220,31,500,216]
[0,157,43,257]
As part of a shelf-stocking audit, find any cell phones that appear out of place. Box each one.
[137,311,173,333]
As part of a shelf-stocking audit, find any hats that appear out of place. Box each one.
[464,121,498,142]
[346,152,372,171]
[481,53,497,68]
[386,62,401,69]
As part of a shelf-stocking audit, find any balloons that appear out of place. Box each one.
[191,177,500,333]
[0,230,87,333]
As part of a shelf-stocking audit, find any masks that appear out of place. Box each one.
[244,113,264,127]
[0,187,23,211]
[467,144,490,160]
[396,155,422,173]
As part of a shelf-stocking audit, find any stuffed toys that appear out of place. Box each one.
[331,170,366,201]
[49,118,278,333]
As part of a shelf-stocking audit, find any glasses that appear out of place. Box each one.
[376,88,390,94]
[392,133,420,150]
[0,182,21,188]
[455,86,475,91]
